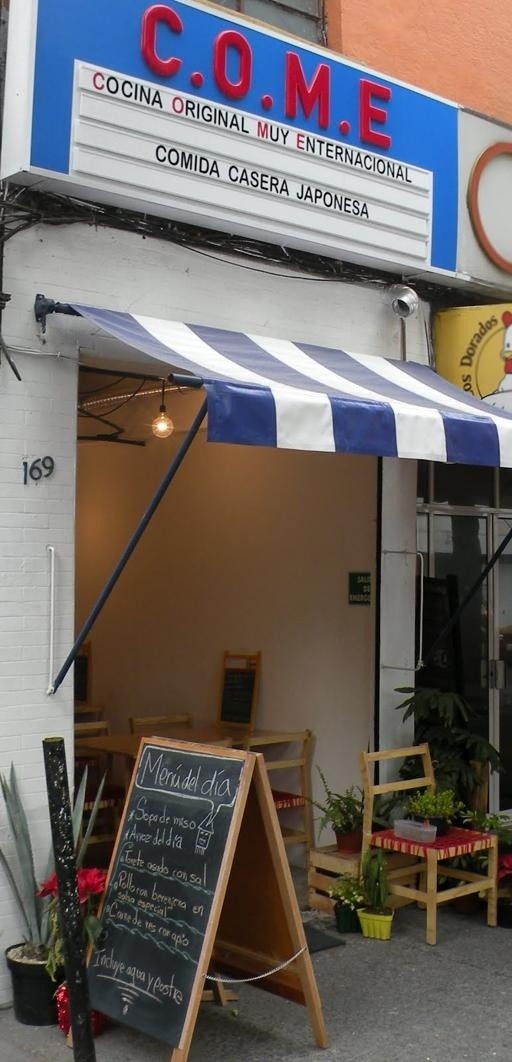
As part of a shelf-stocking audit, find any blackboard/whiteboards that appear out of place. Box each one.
[215,663,261,731]
[85,736,256,1049]
[74,652,93,701]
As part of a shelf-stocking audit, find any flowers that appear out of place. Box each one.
[497,853,512,898]
[328,872,359,906]
[35,867,107,1000]
[398,789,466,817]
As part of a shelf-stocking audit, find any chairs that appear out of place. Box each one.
[360,744,498,947]
[74,707,314,873]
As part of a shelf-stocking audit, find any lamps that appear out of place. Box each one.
[151,378,173,438]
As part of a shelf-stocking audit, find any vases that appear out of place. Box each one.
[499,898,512,928]
[412,814,450,837]
[56,980,106,1038]
[334,904,360,932]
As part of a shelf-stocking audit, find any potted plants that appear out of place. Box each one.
[0,761,107,1027]
[299,765,364,853]
[356,847,395,941]
